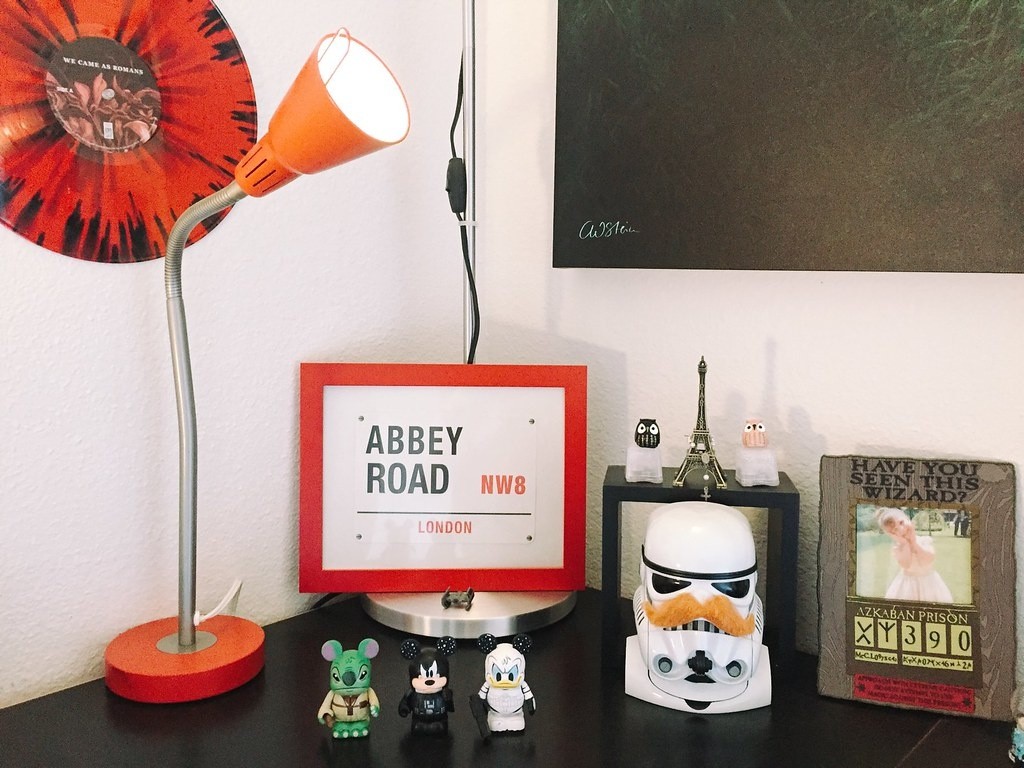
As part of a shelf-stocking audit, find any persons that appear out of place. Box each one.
[874,507,955,603]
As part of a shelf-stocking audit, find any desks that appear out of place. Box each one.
[600,464,800,676]
[0,586,1024,768]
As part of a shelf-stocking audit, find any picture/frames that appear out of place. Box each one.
[815,455,1019,722]
[300,362,588,594]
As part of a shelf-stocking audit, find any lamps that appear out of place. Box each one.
[105,31,411,705]
